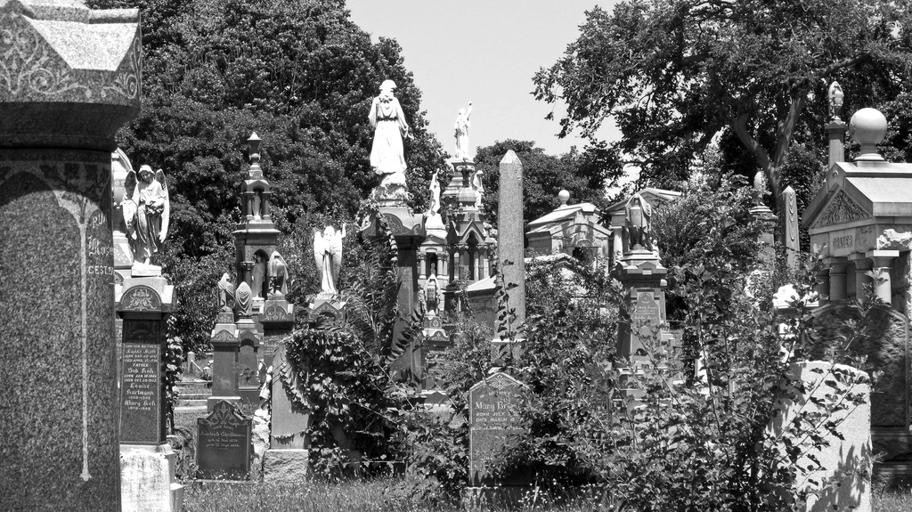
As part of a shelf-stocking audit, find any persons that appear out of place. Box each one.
[427,168,443,214]
[130,163,166,267]
[452,99,474,155]
[316,222,350,296]
[367,77,411,178]
